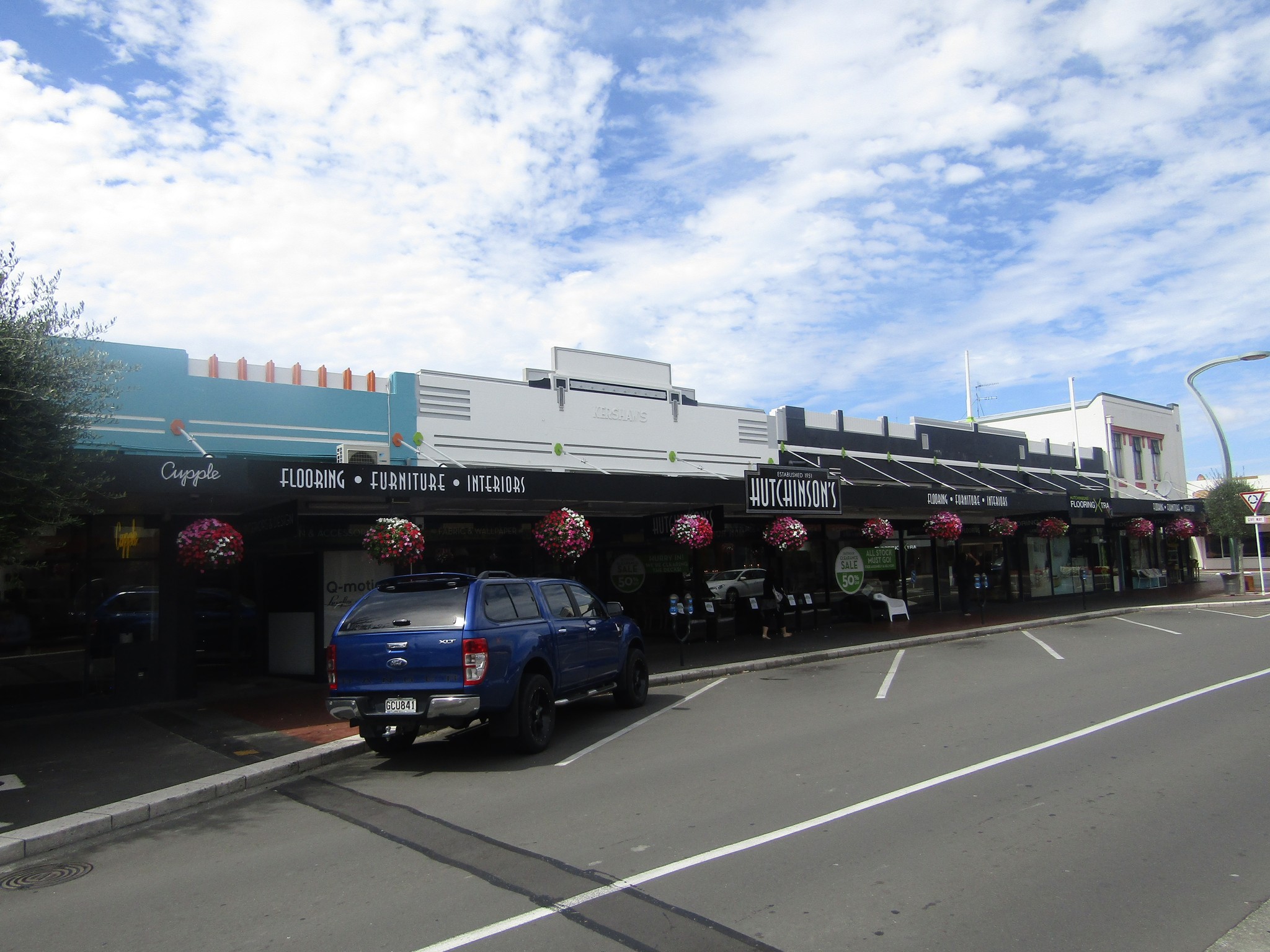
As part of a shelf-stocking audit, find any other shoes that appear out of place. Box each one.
[783,633,792,637]
[761,635,771,639]
[964,613,971,616]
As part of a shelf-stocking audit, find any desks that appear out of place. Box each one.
[1191,567,1201,582]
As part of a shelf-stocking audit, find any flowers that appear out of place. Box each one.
[362,516,425,567]
[670,513,713,549]
[761,516,809,553]
[1035,517,1069,541]
[923,511,963,544]
[175,517,245,574]
[531,507,594,564]
[1125,517,1155,539]
[987,518,1019,540]
[860,517,894,543]
[1164,516,1198,540]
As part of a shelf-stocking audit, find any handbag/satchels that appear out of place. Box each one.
[772,585,783,603]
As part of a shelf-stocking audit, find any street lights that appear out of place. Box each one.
[1181,350,1270,597]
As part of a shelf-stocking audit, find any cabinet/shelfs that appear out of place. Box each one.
[1167,543,1180,570]
[1073,570,1094,593]
[1094,574,1112,591]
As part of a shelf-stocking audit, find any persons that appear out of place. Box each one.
[958,553,980,616]
[762,567,792,640]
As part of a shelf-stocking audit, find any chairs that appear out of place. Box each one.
[873,593,910,622]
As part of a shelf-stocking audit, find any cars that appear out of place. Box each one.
[705,567,767,605]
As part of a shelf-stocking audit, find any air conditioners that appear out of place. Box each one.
[336,444,390,465]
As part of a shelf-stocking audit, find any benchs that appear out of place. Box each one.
[1136,568,1167,589]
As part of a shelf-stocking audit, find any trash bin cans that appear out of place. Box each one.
[1220,572,1241,596]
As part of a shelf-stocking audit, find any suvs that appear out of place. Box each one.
[323,569,650,760]
[61,577,174,649]
